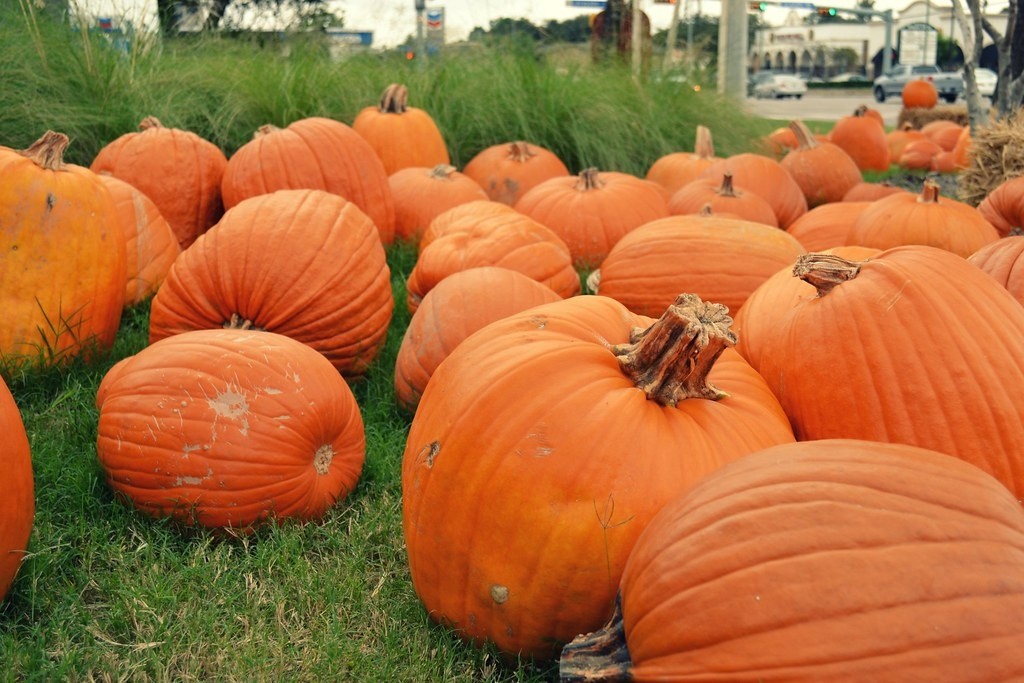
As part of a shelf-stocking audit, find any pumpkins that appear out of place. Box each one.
[0,82,1024,683]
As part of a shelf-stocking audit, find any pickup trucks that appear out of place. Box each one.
[874,61,963,103]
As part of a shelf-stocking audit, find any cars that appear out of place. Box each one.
[755,75,805,99]
[958,67,999,96]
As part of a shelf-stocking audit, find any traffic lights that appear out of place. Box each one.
[406,51,414,60]
[758,1,768,11]
[828,8,836,17]
